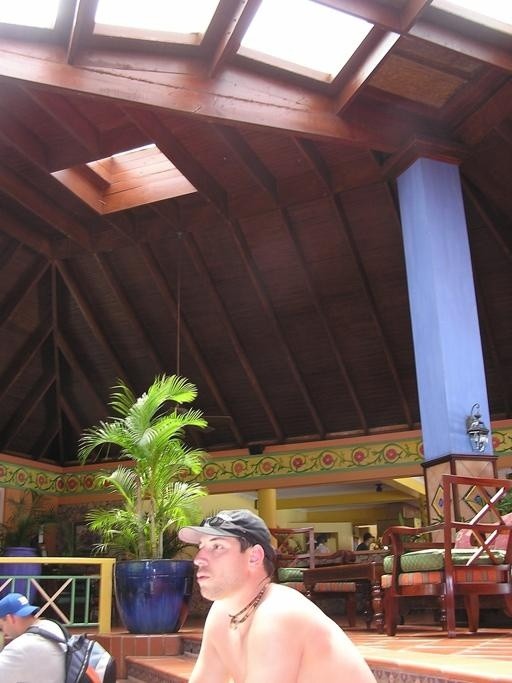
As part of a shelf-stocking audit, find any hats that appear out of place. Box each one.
[177,508,275,560]
[0,592,40,619]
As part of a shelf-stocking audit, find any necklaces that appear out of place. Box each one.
[229,581,266,632]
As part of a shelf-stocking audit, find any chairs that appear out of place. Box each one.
[265,524,356,627]
[375,473,512,638]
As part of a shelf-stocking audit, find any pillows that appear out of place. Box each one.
[454,512,512,550]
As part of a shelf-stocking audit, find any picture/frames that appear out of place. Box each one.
[71,521,95,556]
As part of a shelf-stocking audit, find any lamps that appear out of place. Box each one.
[466,403,489,453]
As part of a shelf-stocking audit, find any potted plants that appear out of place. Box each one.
[1,486,75,607]
[79,372,222,635]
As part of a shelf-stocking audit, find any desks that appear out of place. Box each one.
[299,561,385,634]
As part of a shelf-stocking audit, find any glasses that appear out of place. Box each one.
[197,516,226,527]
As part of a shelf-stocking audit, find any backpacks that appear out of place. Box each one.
[25,618,117,682]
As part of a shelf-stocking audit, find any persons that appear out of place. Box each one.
[172,509,379,682]
[315,533,330,551]
[1,591,72,683]
[357,532,376,550]
